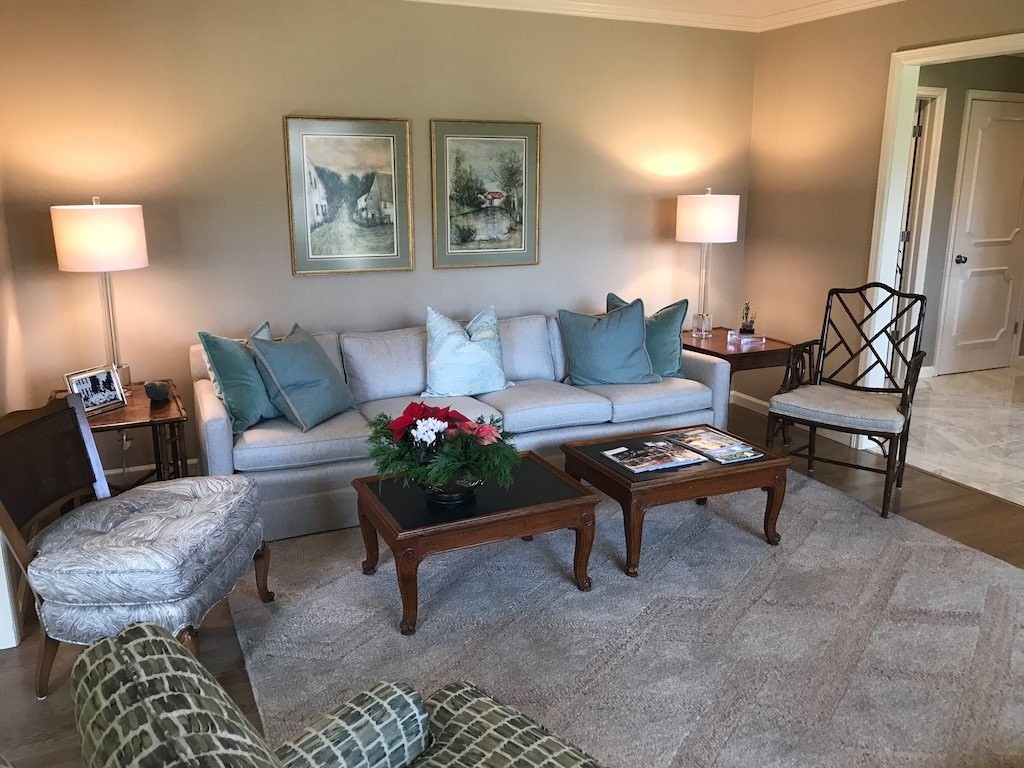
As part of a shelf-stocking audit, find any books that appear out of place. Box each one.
[599,439,708,474]
[663,426,764,466]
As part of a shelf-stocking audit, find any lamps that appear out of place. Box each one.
[675,187,739,339]
[50,196,150,384]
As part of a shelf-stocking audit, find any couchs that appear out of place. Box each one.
[189,314,731,540]
[71,622,606,768]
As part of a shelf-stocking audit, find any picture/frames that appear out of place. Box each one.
[62,363,128,418]
[283,115,414,276]
[430,119,541,269]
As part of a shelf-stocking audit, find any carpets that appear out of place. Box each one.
[228,467,1024,768]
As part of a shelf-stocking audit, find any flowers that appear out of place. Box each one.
[365,400,522,493]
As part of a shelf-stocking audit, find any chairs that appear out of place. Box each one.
[0,394,274,700]
[760,282,926,519]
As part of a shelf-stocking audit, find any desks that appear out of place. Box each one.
[89,379,187,482]
[681,326,798,446]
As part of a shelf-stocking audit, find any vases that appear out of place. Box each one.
[411,466,485,505]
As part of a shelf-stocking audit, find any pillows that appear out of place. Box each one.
[198,321,358,432]
[557,293,688,386]
[419,305,515,397]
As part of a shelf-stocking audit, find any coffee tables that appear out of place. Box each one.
[351,450,603,634]
[559,423,792,576]
